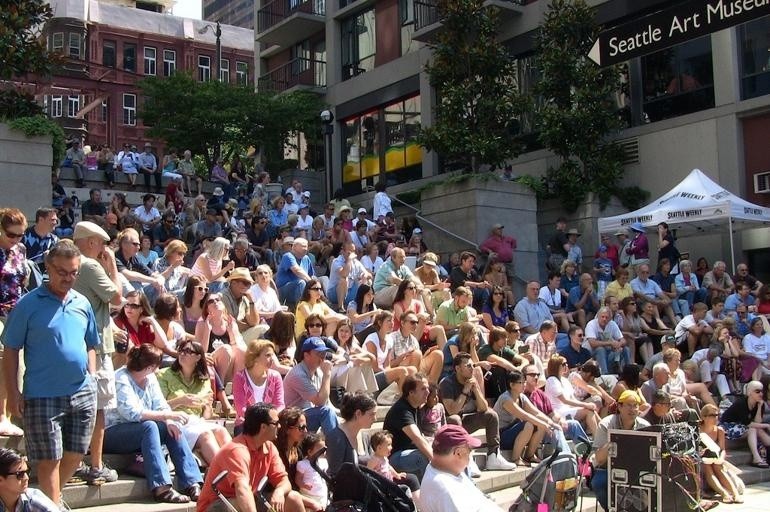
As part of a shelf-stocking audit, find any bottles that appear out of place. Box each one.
[116,327,129,353]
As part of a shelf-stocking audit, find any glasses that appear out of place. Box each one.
[4,466,31,480]
[657,403,672,407]
[208,297,222,303]
[737,312,746,314]
[621,277,629,280]
[132,147,137,149]
[52,264,80,279]
[308,288,323,290]
[752,389,765,393]
[740,269,748,271]
[268,421,280,427]
[175,251,187,256]
[131,243,141,246]
[511,329,521,332]
[576,334,585,337]
[527,373,540,377]
[125,303,141,309]
[199,199,205,201]
[493,292,503,295]
[3,225,25,238]
[640,271,649,274]
[516,381,527,384]
[305,197,309,199]
[166,220,175,223]
[145,146,151,149]
[628,303,637,306]
[405,320,419,324]
[178,348,197,355]
[302,337,330,351]
[259,222,267,224]
[704,414,718,417]
[622,404,639,409]
[224,248,229,251]
[462,364,476,368]
[234,248,246,252]
[292,425,306,431]
[102,146,110,147]
[124,146,130,147]
[309,323,322,327]
[194,286,209,292]
[406,287,416,290]
[336,223,344,225]
[560,362,568,367]
[279,202,285,204]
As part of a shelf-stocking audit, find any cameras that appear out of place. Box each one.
[484,371,492,381]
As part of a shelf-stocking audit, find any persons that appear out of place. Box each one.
[18,206,61,296]
[0,447,63,512]
[1,237,101,512]
[0,206,31,441]
[66,136,256,198]
[514,216,770,512]
[52,168,561,511]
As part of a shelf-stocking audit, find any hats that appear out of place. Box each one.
[618,390,641,404]
[423,252,437,266]
[432,424,482,451]
[304,191,310,197]
[72,138,80,144]
[566,229,581,235]
[660,334,679,344]
[281,236,294,243]
[340,206,352,212]
[630,223,646,233]
[614,228,629,236]
[492,224,504,229]
[358,207,366,213]
[599,245,608,251]
[227,267,254,283]
[214,187,224,195]
[205,209,216,215]
[413,228,421,234]
[73,221,110,241]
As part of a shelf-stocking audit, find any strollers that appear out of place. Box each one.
[507,436,593,512]
[310,446,415,512]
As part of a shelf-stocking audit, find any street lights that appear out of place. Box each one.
[197,20,222,82]
[319,108,338,203]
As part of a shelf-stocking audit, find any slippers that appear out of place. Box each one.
[752,460,769,468]
[524,454,542,463]
[511,457,531,466]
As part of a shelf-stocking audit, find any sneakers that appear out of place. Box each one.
[87,463,118,485]
[721,495,734,502]
[188,193,192,197]
[1,423,24,437]
[57,500,71,512]
[719,398,733,408]
[78,183,83,187]
[84,183,87,187]
[109,185,114,189]
[732,389,737,395]
[110,181,114,185]
[737,388,742,396]
[735,494,744,503]
[486,448,517,470]
[339,307,346,312]
[467,450,481,478]
[131,185,136,190]
[129,185,133,189]
[65,461,91,483]
[221,406,236,419]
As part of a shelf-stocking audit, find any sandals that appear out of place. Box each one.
[187,486,202,502]
[155,488,191,502]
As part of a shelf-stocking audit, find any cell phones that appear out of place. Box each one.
[519,344,530,355]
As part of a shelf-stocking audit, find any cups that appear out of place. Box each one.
[280,304,289,313]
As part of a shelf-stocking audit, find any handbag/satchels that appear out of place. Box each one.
[330,386,346,409]
[126,450,145,477]
[377,381,400,406]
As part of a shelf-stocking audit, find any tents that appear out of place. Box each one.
[597,169,770,274]
